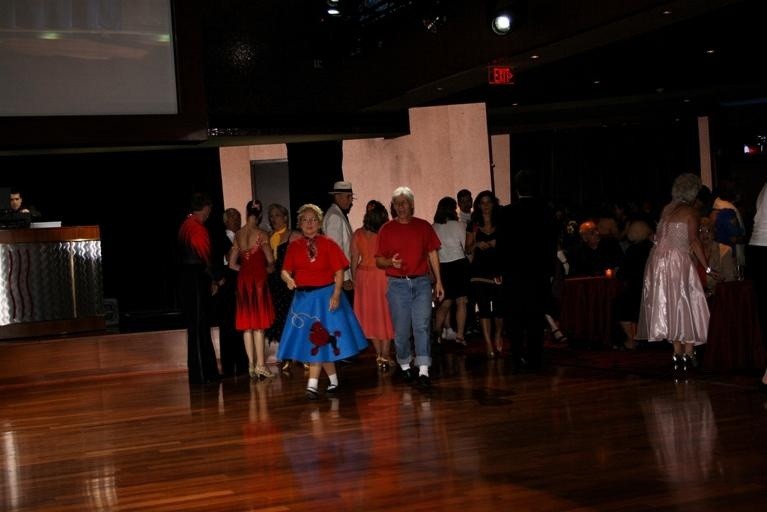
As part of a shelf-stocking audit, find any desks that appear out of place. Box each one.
[558,273,624,346]
[695,276,763,374]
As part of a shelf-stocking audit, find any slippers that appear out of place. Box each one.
[613,343,634,352]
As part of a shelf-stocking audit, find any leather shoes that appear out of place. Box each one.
[325,384,341,394]
[306,386,319,404]
[402,369,431,387]
[487,345,502,365]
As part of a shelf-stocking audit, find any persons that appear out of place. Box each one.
[0,191,32,229]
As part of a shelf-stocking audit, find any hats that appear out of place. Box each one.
[328,181,357,195]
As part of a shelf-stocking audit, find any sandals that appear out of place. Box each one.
[552,329,567,342]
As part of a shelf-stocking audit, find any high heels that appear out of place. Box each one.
[377,354,396,368]
[248,367,277,379]
[671,353,681,383]
[682,354,703,383]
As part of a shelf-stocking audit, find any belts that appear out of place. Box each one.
[390,275,420,279]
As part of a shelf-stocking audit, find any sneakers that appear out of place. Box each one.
[441,327,467,346]
